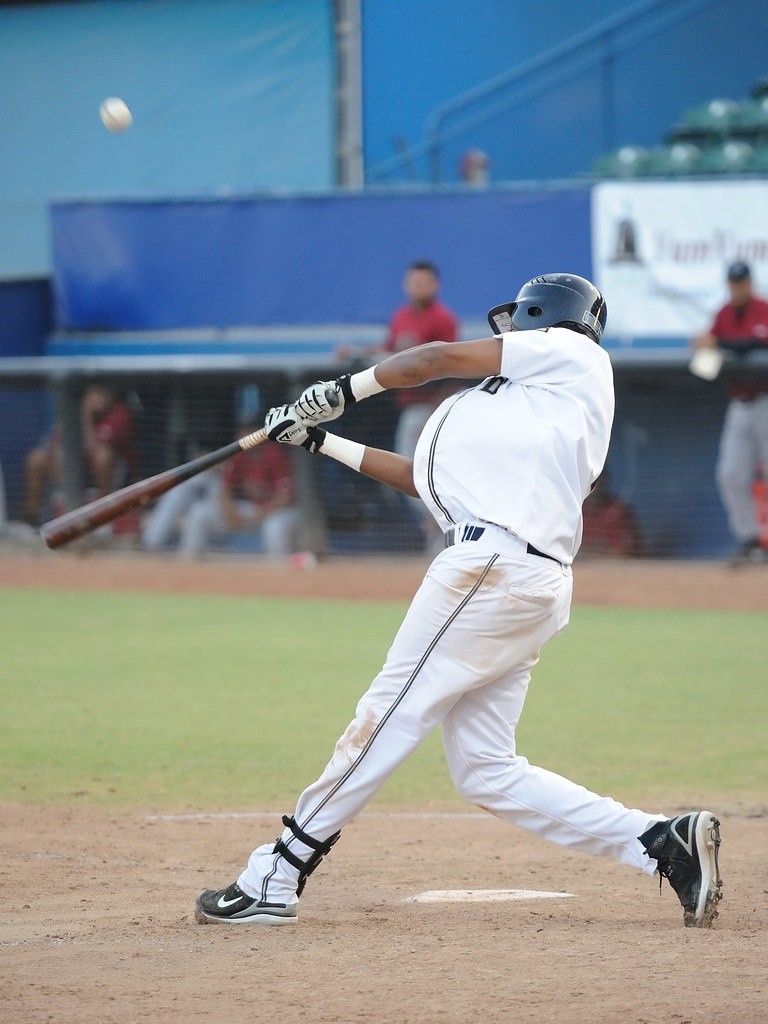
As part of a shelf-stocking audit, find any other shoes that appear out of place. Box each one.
[727,539,762,567]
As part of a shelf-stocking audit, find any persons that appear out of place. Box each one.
[691,258,768,567]
[335,258,457,550]
[461,150,489,182]
[193,273,721,926]
[140,407,309,565]
[22,374,134,546]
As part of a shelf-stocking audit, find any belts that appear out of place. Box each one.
[445,525,567,565]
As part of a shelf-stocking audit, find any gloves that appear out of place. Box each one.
[293,380,356,427]
[263,403,326,456]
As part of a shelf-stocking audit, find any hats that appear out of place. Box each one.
[727,260,749,281]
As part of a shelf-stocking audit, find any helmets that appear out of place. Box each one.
[486,272,607,344]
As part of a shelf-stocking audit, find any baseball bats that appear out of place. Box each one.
[38,389,340,551]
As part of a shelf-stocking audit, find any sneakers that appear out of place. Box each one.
[194,881,298,925]
[637,810,722,928]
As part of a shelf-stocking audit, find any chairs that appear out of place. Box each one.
[587,84,767,177]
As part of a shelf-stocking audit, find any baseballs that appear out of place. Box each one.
[98,96,133,131]
